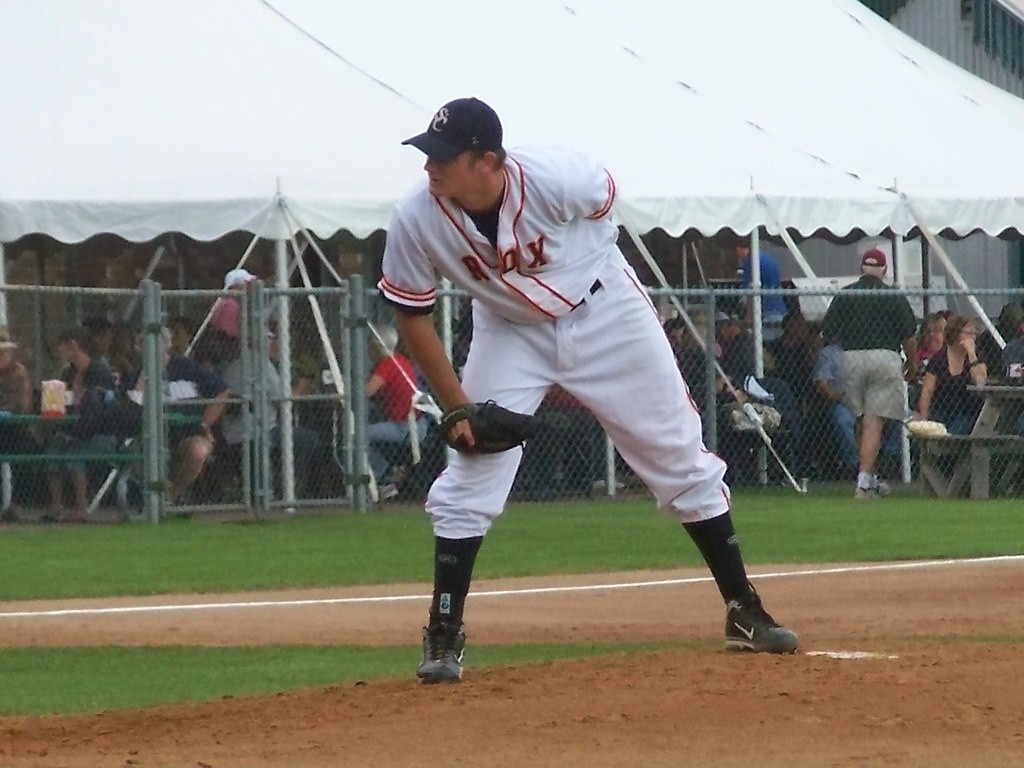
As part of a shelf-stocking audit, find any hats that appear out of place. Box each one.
[0,325,16,348]
[401,97,502,157]
[224,269,256,290]
[863,251,885,266]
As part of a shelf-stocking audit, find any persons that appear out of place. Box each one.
[0,237,1023,500]
[378,98,799,686]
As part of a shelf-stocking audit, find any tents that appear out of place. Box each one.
[0,0,1024,507]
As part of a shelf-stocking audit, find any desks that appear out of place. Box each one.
[0,413,203,512]
[944,384,1024,496]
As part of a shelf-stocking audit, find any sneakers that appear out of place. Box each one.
[855,483,892,498]
[416,623,466,681]
[725,601,799,653]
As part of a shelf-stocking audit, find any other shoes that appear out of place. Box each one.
[591,479,626,489]
[38,508,95,523]
[379,484,398,499]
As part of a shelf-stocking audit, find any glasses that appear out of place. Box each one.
[1,334,15,342]
[962,330,978,337]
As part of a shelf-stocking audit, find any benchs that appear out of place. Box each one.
[910,433,1024,501]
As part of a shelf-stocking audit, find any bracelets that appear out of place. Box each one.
[971,359,980,367]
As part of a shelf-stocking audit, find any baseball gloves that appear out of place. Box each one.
[441,401,534,457]
